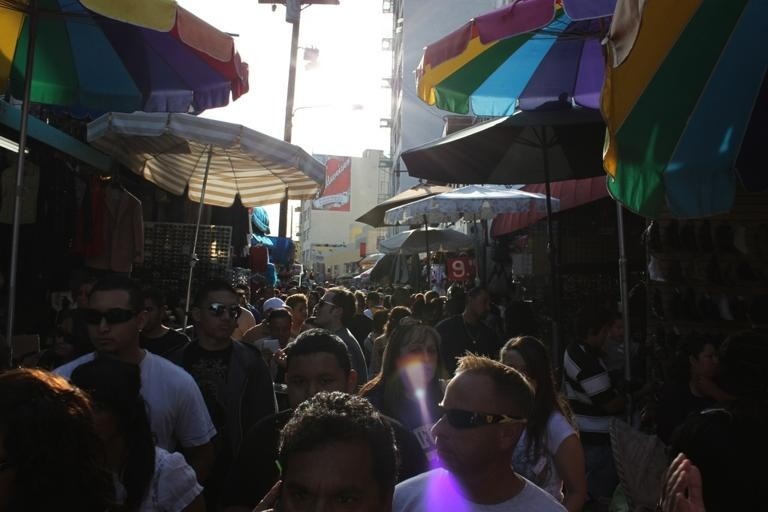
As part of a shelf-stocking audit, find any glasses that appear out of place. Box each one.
[318,298,341,306]
[198,304,241,321]
[433,407,527,429]
[86,307,139,325]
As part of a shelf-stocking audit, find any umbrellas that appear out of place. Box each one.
[415,1,683,381]
[376,227,466,288]
[355,181,455,289]
[384,183,561,283]
[87,109,326,334]
[0,0,251,367]
[400,116,606,322]
[601,0,767,222]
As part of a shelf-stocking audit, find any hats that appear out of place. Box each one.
[262,297,290,313]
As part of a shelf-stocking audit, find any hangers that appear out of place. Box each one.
[106,175,125,191]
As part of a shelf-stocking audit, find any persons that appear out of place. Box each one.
[1,267,767,511]
